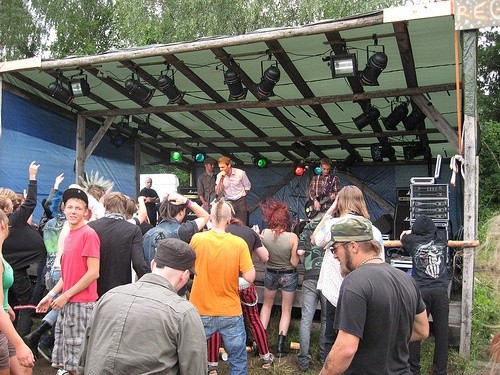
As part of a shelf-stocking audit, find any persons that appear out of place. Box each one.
[318,216,429,375]
[197,157,218,213]
[36,188,101,375]
[297,199,328,373]
[309,158,341,217]
[251,195,304,356]
[312,184,386,362]
[88,192,153,301]
[0,160,113,363]
[122,191,274,375]
[0,209,36,375]
[214,157,251,225]
[401,215,448,375]
[79,238,210,375]
[140,177,161,228]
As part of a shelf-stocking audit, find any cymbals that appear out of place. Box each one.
[290,194,307,198]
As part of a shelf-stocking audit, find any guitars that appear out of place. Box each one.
[305,192,337,219]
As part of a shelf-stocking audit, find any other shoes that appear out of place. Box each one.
[37,342,52,362]
[299,363,308,371]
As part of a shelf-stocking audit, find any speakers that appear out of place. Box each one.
[392,202,411,241]
[177,187,203,223]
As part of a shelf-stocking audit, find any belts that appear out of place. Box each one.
[267,268,295,274]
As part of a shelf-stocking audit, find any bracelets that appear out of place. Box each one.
[49,291,56,297]
[185,200,192,208]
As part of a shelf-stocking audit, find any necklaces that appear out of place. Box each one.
[360,257,382,266]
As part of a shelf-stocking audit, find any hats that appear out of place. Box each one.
[204,158,217,164]
[63,188,88,208]
[218,157,231,164]
[322,216,372,250]
[155,238,196,275]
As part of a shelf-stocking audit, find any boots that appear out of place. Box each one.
[24,320,51,358]
[276,331,287,357]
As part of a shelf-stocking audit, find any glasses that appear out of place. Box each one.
[330,243,346,253]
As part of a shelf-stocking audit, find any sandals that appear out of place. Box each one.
[208,366,217,375]
[261,354,274,368]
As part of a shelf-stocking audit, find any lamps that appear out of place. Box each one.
[379,96,409,131]
[47,71,74,105]
[160,146,182,162]
[321,42,357,79]
[192,148,206,163]
[69,69,90,97]
[233,158,245,170]
[355,34,388,87]
[334,151,356,173]
[294,162,322,177]
[351,100,382,133]
[290,142,311,159]
[253,48,281,102]
[403,140,426,161]
[370,140,394,162]
[156,62,187,104]
[124,66,156,106]
[251,154,268,169]
[138,113,163,139]
[221,57,249,101]
[107,115,140,149]
[402,104,426,131]
